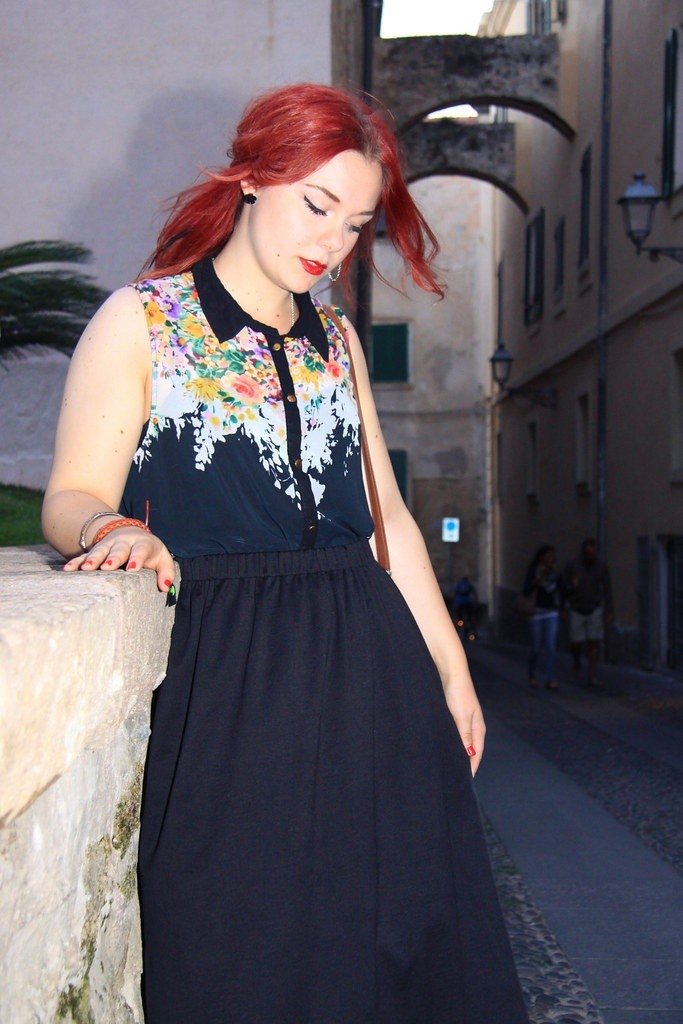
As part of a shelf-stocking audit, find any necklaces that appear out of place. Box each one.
[210,257,294,328]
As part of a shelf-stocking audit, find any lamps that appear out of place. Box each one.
[490,342,558,407]
[617,171,683,265]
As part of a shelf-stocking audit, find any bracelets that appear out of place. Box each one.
[93,517,152,547]
[80,512,127,554]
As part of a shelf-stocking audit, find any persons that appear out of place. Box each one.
[561,536,615,694]
[520,545,569,691]
[41,78,534,1024]
